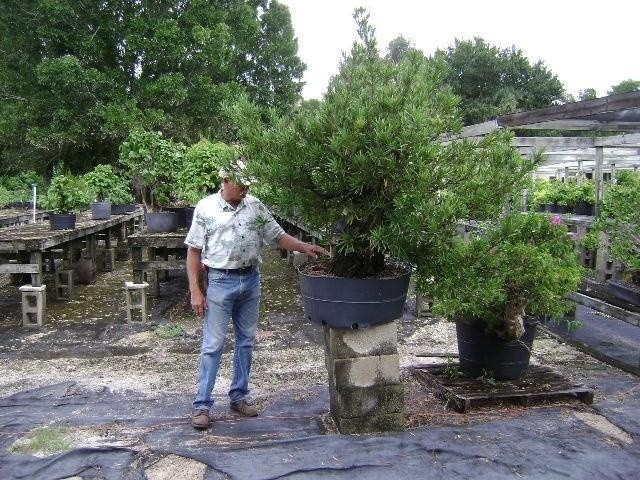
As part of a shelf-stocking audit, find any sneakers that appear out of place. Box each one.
[230,401,259,417]
[190,409,209,428]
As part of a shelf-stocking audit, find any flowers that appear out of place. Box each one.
[415,209,583,340]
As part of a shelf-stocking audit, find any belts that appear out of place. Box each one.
[219,268,251,274]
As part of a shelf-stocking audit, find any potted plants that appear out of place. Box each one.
[216,4,548,329]
[47,127,215,230]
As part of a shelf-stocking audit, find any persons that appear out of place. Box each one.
[184,159,330,429]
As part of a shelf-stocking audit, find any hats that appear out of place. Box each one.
[218,159,259,187]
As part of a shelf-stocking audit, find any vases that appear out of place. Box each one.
[454,314,537,379]
